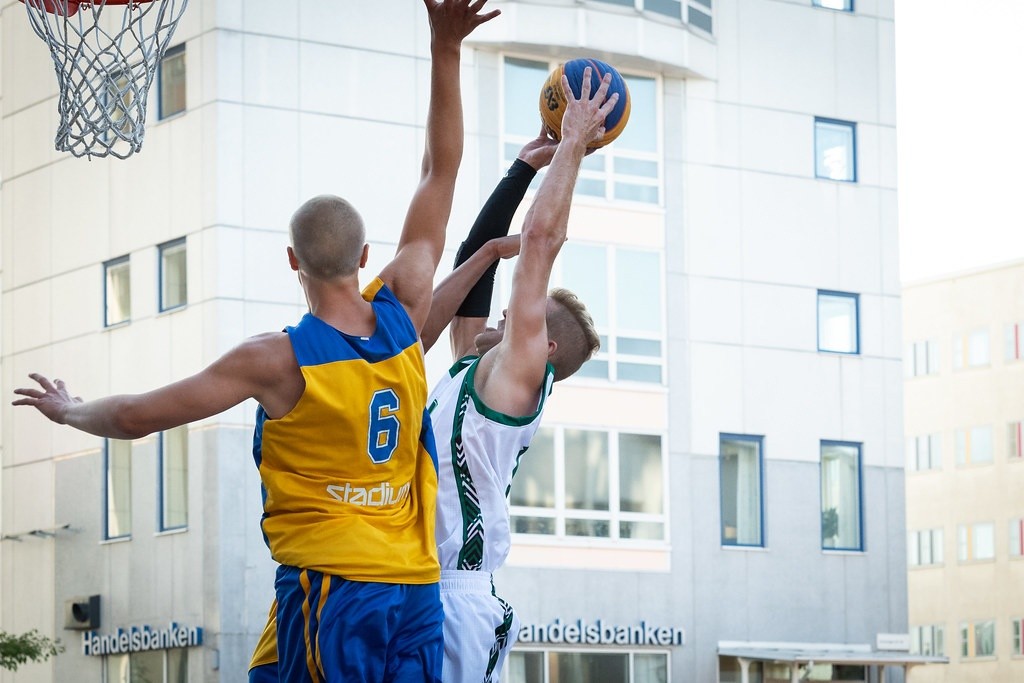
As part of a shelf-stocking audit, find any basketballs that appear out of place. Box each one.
[539,57,632,150]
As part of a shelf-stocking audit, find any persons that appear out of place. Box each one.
[12,0,620,683]
